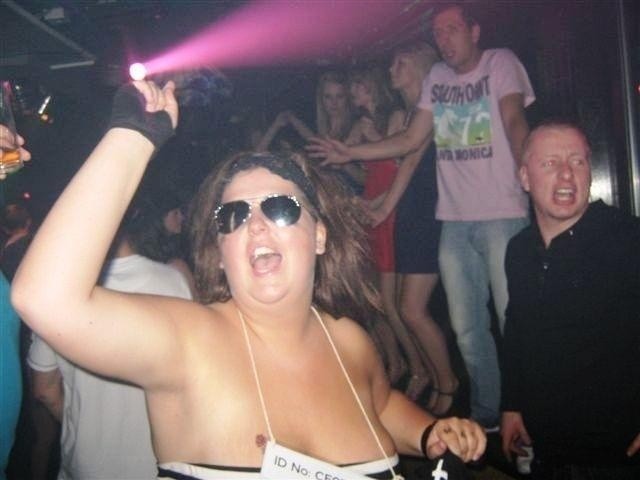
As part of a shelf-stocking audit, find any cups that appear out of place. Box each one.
[1,87,26,176]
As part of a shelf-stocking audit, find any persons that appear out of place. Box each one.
[1,1,639,480]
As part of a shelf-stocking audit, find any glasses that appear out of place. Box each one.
[213,193,318,234]
